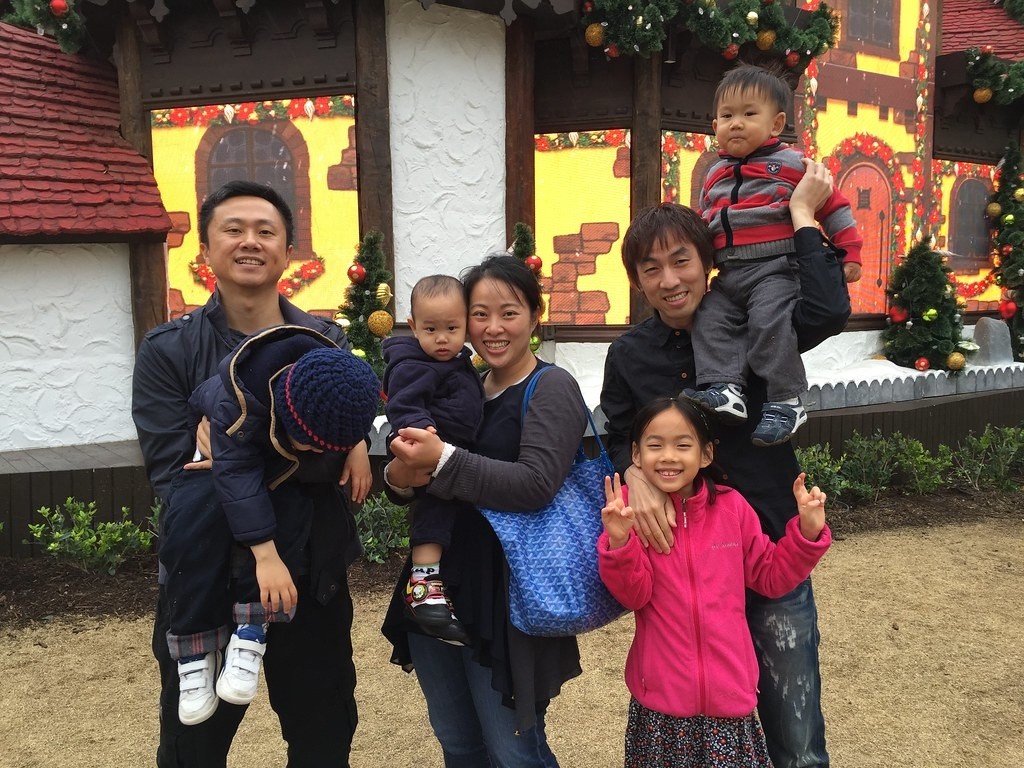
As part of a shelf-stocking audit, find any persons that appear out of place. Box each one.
[595,398,833,768]
[129,179,590,768]
[678,66,863,447]
[601,204,852,768]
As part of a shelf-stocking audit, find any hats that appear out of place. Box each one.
[273,346,381,455]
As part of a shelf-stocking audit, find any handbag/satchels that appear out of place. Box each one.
[478,365,633,635]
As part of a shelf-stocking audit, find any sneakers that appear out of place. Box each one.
[176,649,222,726]
[404,604,468,646]
[680,381,749,422]
[751,395,808,446]
[214,621,270,705]
[400,574,452,627]
